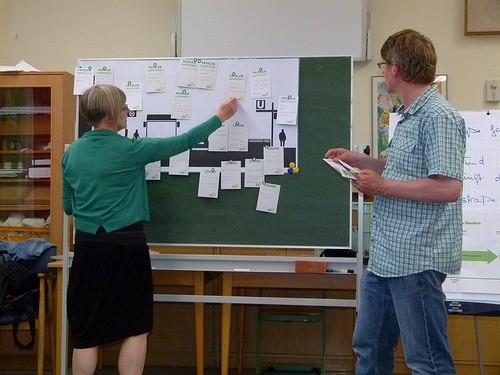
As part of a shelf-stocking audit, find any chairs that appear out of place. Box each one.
[255,289,327,375]
[0,237,58,375]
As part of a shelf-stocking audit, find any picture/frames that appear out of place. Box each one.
[372,74,448,159]
[464,0,500,36]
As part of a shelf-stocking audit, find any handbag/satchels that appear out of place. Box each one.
[0,238,58,349]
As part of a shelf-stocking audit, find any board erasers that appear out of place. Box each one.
[294,259,327,273]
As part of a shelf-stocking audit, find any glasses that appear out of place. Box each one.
[122,104,128,111]
[377,60,386,69]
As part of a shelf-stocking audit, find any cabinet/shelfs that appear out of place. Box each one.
[0,70,77,375]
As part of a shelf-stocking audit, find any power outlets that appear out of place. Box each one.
[486,80,500,102]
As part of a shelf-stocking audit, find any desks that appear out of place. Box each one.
[47,260,358,375]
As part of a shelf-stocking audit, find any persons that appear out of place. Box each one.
[60,83,237,375]
[325,29,463,375]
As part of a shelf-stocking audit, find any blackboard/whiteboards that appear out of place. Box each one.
[70,55,354,251]
[176,1,367,61]
[386,109,500,295]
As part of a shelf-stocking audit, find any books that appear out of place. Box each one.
[0,105,51,227]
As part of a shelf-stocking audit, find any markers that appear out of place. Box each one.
[327,269,355,273]
